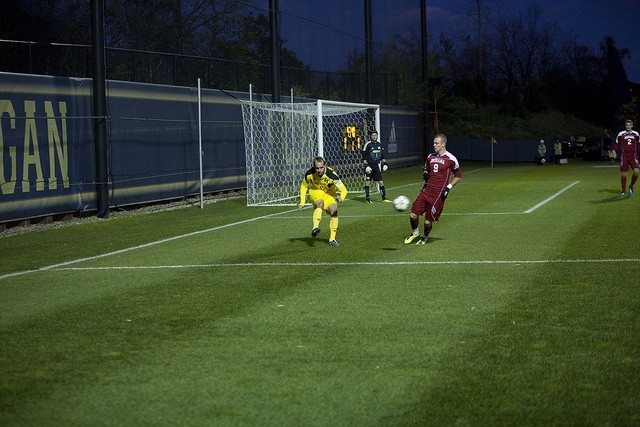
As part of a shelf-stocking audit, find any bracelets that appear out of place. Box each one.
[445,184,452,189]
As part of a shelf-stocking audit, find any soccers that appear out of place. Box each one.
[393,194,410,211]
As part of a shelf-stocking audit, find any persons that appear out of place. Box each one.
[600,128,615,164]
[403,134,462,245]
[537,140,546,165]
[298,156,348,246]
[612,120,640,198]
[553,139,563,165]
[362,130,392,203]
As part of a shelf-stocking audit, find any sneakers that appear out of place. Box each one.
[415,235,429,245]
[328,240,339,247]
[312,228,320,237]
[620,191,625,195]
[404,233,420,245]
[382,200,393,203]
[366,201,373,204]
[629,186,634,196]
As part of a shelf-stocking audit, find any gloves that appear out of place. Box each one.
[442,187,449,199]
[423,173,429,183]
[383,165,388,172]
[365,166,372,174]
